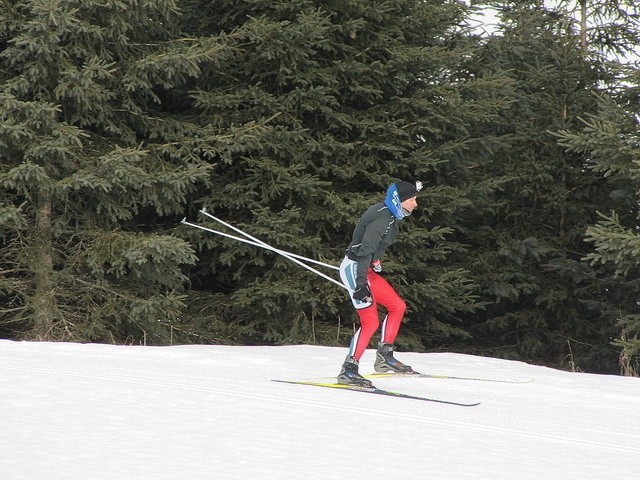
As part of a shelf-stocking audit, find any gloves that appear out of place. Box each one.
[374,265,382,274]
[354,284,371,303]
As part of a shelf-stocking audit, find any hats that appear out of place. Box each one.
[396,180,417,202]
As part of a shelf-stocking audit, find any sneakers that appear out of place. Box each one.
[374,358,412,372]
[337,370,372,387]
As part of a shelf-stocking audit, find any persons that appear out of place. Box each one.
[337,180,419,387]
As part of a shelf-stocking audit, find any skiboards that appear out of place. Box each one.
[272,372,538,406]
